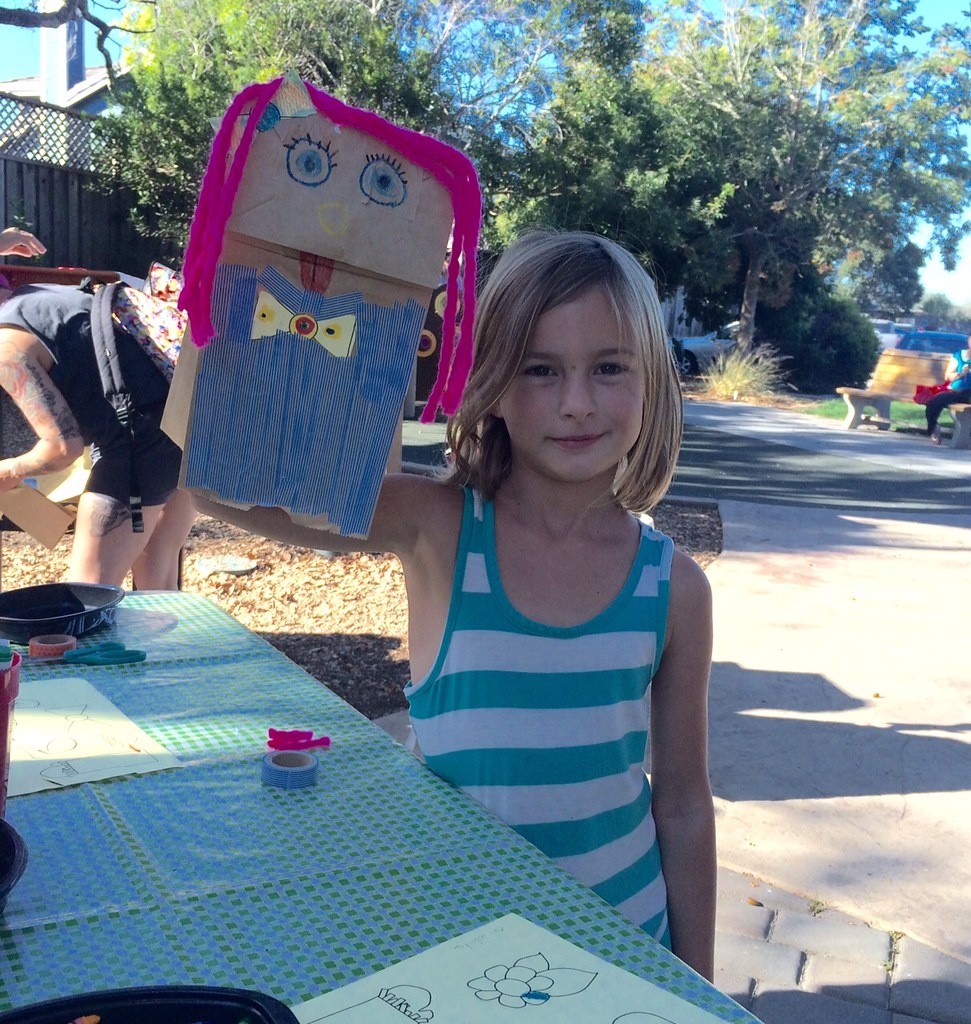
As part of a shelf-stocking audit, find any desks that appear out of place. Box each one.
[0,590,763,1023]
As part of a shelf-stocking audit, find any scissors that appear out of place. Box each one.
[20,641,147,665]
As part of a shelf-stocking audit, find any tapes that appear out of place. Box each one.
[261,750,320,791]
[28,633,77,658]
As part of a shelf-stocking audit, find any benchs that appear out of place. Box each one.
[837,347,971,449]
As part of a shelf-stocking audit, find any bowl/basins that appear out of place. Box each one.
[0,819,29,903]
[0,985,299,1023]
[0,582,125,643]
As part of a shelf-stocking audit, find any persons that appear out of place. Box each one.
[0,227,197,592]
[190,230,716,981]
[926,334,971,444]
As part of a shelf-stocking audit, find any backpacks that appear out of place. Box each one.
[89,259,189,449]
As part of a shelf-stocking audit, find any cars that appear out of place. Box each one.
[859,310,969,357]
[671,322,742,377]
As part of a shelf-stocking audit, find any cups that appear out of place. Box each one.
[0,651,22,819]
